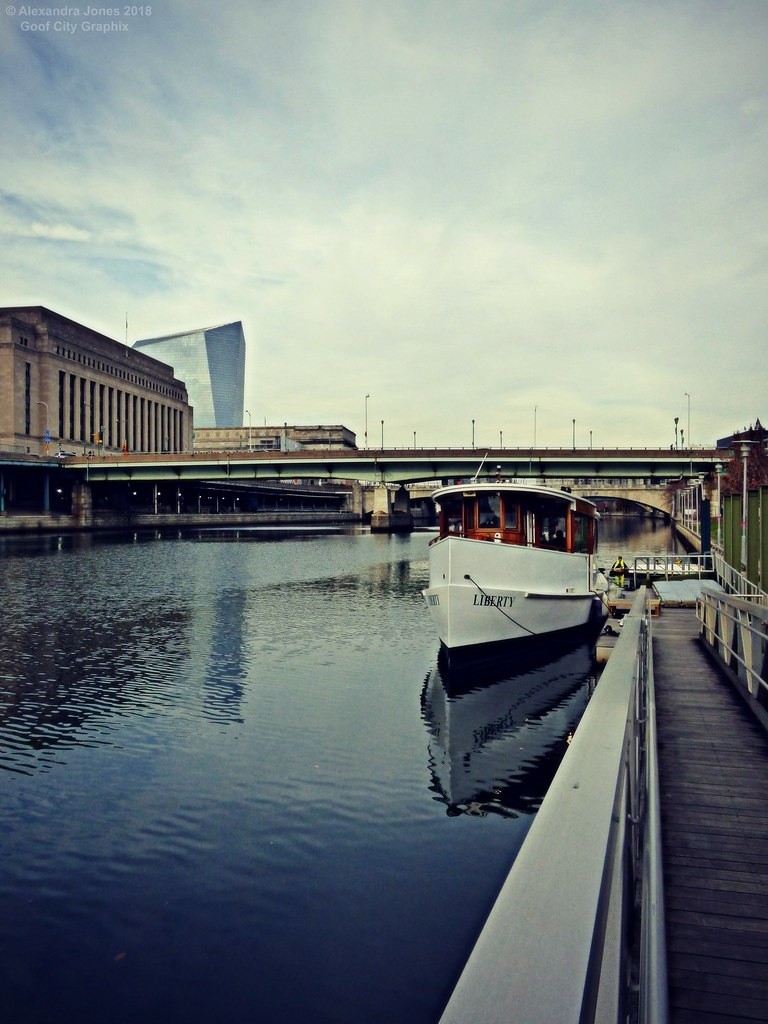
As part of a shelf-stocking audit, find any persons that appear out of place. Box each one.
[486,510,500,528]
[554,530,566,550]
[610,556,629,589]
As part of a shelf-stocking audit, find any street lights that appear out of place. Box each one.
[472,419,475,449]
[245,409,253,452]
[500,431,503,448]
[711,460,728,551]
[572,419,576,448]
[363,393,371,451]
[381,421,384,451]
[533,403,540,449]
[413,431,416,449]
[733,440,759,595]
[699,472,705,501]
[684,391,692,449]
[36,400,50,456]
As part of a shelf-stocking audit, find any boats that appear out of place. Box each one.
[419,479,611,672]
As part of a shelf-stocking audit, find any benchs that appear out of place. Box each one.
[609,599,661,618]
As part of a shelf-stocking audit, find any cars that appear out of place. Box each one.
[53,452,76,459]
